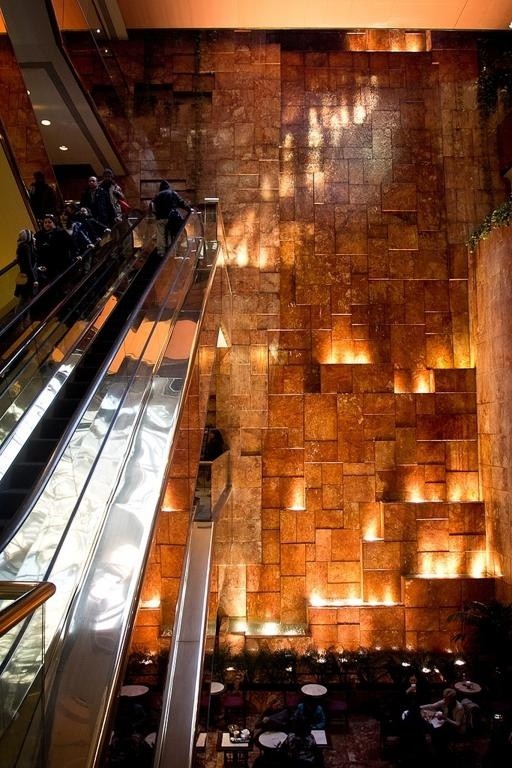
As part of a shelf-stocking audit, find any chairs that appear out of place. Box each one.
[327,683,351,731]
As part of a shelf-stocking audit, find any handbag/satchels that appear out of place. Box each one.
[15,272,28,284]
[168,208,183,232]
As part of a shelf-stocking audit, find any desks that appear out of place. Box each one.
[454,680,483,694]
[119,682,150,697]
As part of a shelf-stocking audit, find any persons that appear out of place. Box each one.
[254,693,293,732]
[289,695,327,735]
[165,205,189,262]
[418,688,468,746]
[148,178,199,258]
[15,168,132,327]
[202,427,225,499]
[398,673,428,744]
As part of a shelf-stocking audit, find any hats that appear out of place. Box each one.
[16,228,31,241]
